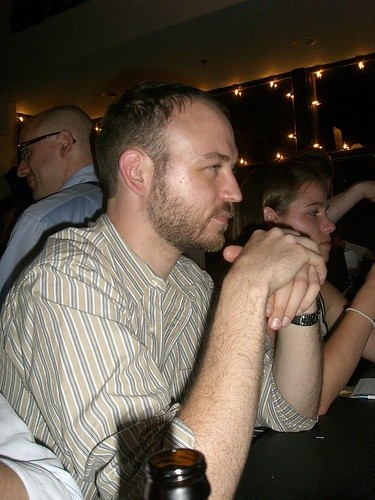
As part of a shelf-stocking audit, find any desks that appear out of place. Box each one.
[236,354,375,499]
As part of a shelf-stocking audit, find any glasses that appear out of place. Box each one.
[17,129,76,160]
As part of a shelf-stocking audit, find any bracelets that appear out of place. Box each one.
[290,296,322,326]
[345,308,374,329]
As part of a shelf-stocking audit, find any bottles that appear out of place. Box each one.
[148,448,211,500]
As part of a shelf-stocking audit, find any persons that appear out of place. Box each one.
[0,104,105,500]
[0,83,328,500]
[211,147,374,416]
